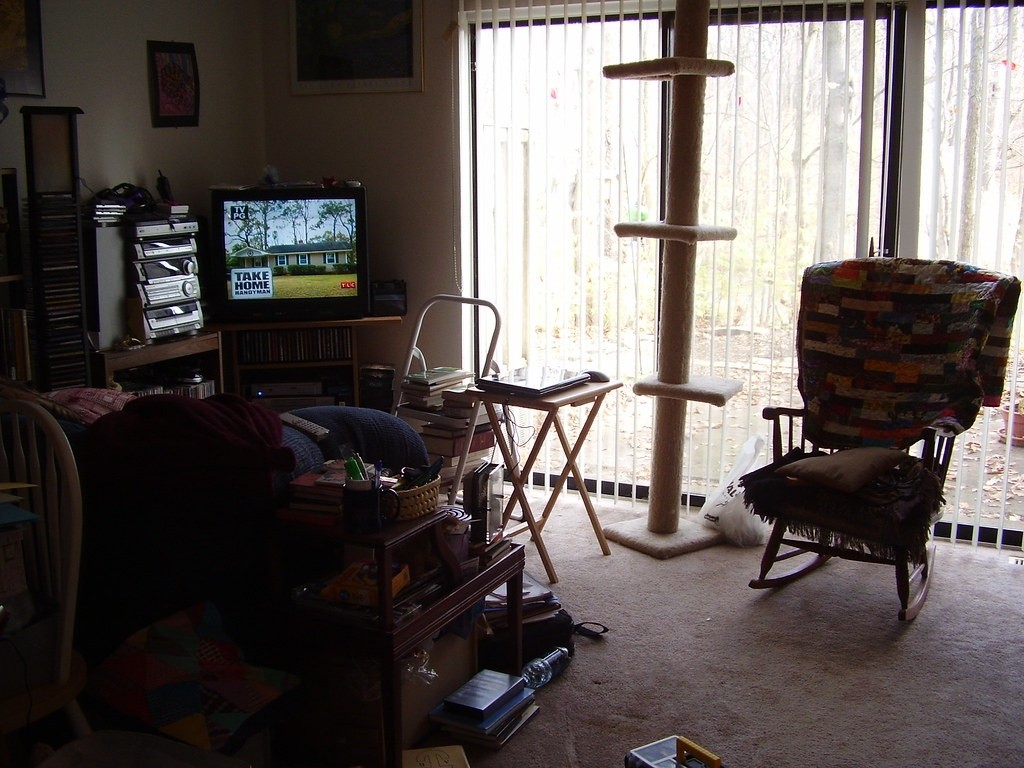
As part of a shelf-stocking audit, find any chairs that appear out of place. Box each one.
[0,398,94,740]
[731,258,1021,622]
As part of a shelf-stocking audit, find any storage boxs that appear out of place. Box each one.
[290,628,474,768]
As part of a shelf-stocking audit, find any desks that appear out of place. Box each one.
[91,328,224,397]
[256,541,523,768]
[200,314,404,415]
[464,381,624,586]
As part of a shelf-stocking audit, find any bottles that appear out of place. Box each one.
[522,645,568,688]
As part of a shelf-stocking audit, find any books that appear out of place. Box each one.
[401,745,470,768]
[429,669,541,748]
[131,378,215,401]
[401,366,495,477]
[277,459,376,525]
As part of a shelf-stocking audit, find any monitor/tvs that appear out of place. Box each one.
[211,186,368,322]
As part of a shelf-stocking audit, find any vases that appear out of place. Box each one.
[1001,405,1024,438]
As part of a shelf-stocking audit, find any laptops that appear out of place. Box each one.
[474,365,591,396]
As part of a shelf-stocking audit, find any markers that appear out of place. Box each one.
[344,456,364,481]
[352,452,369,483]
[374,456,382,491]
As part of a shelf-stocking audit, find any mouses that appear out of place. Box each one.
[583,371,610,382]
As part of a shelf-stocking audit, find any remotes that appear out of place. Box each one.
[344,178,362,188]
[279,412,330,442]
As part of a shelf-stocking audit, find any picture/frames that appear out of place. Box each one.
[147,39,200,128]
[0,0,47,99]
[289,0,425,96]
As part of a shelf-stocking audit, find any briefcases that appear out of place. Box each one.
[624,735,729,768]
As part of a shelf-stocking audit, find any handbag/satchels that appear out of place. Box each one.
[477,610,609,674]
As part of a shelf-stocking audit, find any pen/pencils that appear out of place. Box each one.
[403,456,445,490]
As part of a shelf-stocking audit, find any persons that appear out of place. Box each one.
[352,562,378,585]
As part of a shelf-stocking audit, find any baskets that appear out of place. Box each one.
[390,475,440,521]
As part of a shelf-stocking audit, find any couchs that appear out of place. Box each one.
[0,376,433,700]
